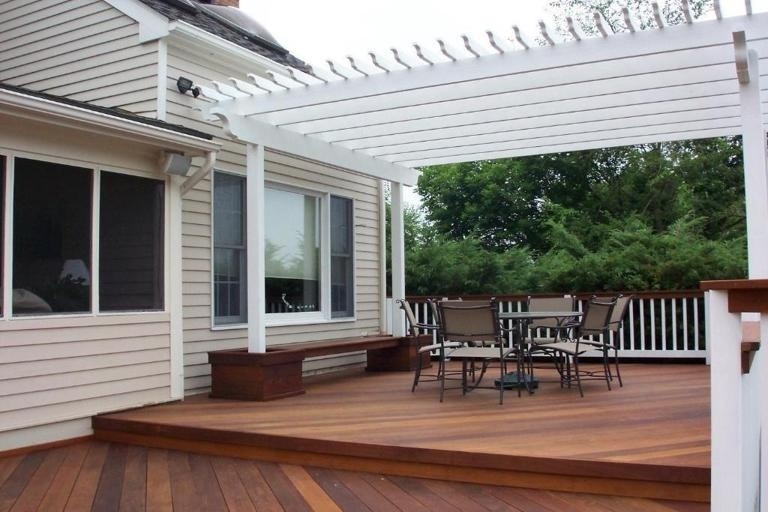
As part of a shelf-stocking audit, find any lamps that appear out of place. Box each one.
[176,76,201,98]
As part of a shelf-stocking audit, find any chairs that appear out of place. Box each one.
[396,291,636,406]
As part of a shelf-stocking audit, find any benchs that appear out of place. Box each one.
[206,334,433,401]
